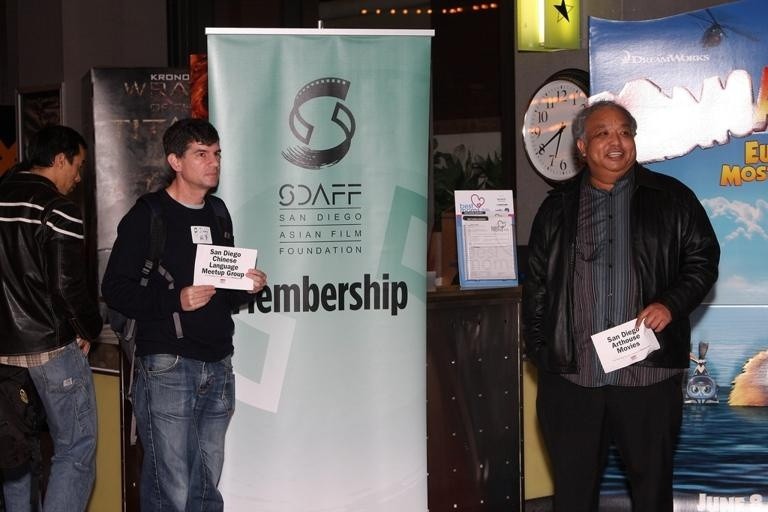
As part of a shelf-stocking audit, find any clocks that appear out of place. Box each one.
[520,68,589,189]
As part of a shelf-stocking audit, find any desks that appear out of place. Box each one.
[119,282,526,512]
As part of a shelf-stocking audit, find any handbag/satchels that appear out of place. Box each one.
[0,365,55,484]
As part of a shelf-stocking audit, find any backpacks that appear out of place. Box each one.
[108,193,229,366]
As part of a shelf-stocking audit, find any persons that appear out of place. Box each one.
[523,102,720,512]
[101,117,267,511]
[0,126,103,511]
